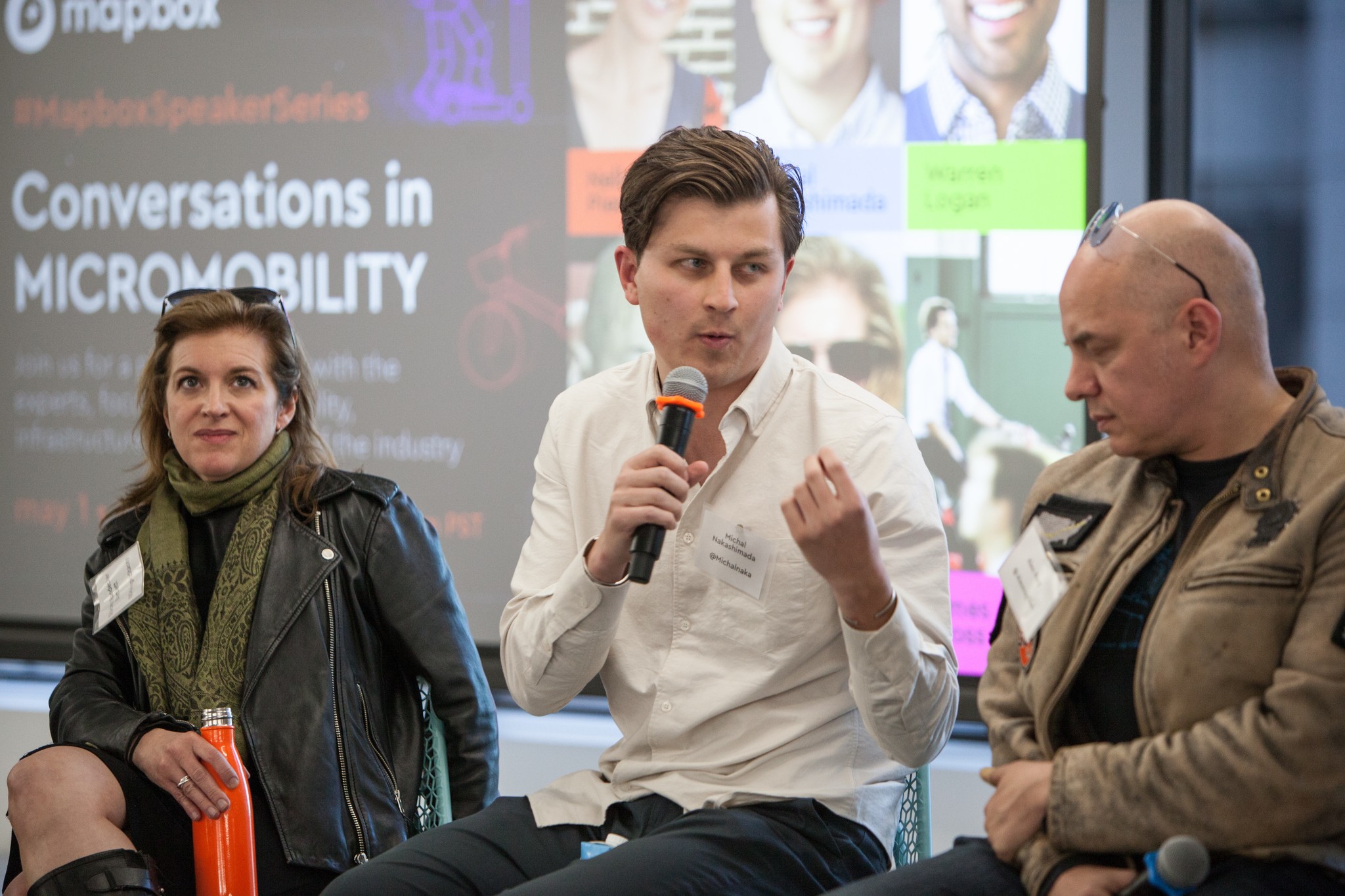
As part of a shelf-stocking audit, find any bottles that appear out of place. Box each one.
[192,707,258,896]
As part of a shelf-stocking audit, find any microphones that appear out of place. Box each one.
[1119,836,1210,896]
[627,365,708,584]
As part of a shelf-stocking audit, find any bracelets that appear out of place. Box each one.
[842,586,896,625]
[583,537,631,586]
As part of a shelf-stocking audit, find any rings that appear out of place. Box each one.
[177,774,190,788]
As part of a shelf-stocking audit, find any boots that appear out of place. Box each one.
[25,848,170,896]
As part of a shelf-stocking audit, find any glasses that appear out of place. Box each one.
[1076,201,1215,307]
[784,339,897,386]
[159,287,297,361]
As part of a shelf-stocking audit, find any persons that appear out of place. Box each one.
[320,125,957,896]
[778,234,1074,578]
[556,0,1088,142]
[1,288,499,896]
[816,199,1345,896]
